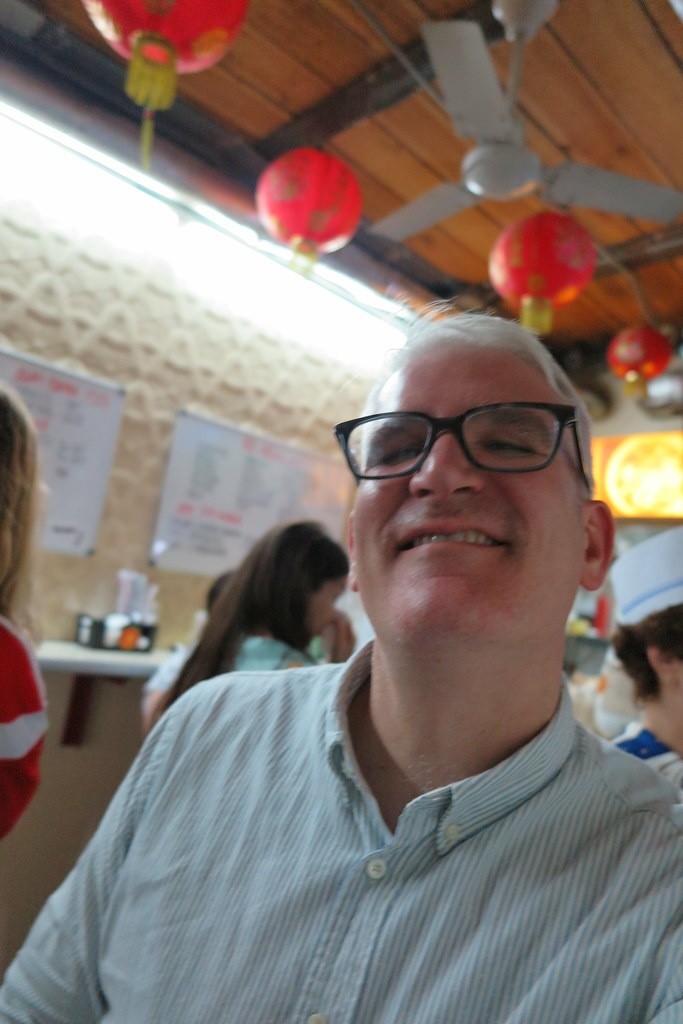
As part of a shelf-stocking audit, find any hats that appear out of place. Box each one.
[610,526,683,627]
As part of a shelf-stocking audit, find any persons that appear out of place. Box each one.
[607,527,683,793]
[142,521,357,732]
[0,385,49,839]
[0,311,683,1024]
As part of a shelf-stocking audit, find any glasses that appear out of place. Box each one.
[331,401,595,496]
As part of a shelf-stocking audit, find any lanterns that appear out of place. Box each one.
[606,322,672,399]
[256,148,363,260]
[487,210,596,336]
[82,0,250,110]
